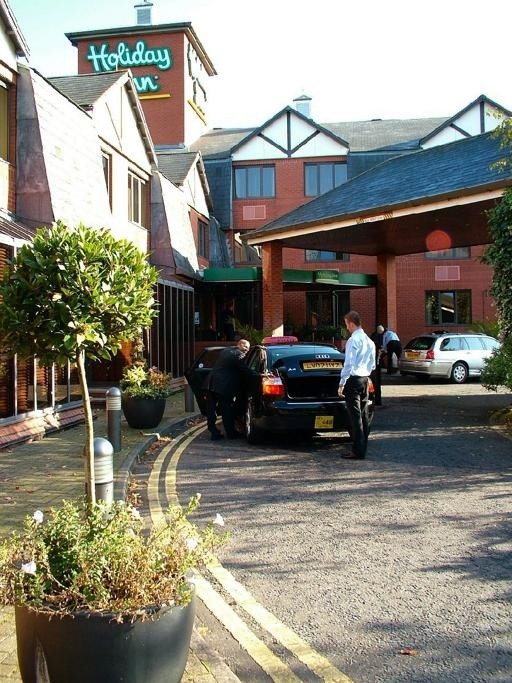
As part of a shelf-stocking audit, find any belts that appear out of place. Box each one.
[350,376,367,378]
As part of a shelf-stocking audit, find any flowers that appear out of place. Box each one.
[1,494,234,626]
[119,365,172,401]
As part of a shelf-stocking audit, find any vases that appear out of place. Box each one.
[17,584,197,683]
[120,399,166,429]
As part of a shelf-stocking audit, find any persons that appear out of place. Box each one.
[369,324,384,366]
[202,338,254,441]
[337,310,376,459]
[383,328,401,376]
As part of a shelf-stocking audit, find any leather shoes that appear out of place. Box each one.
[341,452,367,458]
[211,432,224,441]
[224,431,242,440]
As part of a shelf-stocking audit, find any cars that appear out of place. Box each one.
[184,336,376,445]
[397,332,504,384]
